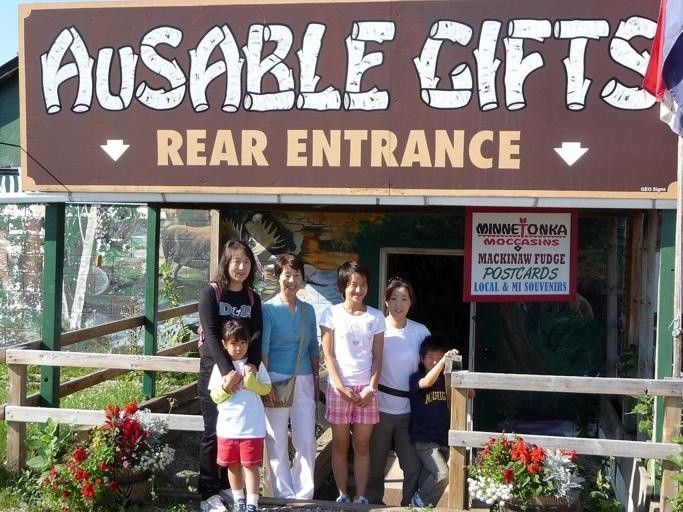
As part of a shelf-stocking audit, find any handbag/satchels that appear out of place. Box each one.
[259,375,296,407]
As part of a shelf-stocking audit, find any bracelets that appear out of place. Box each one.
[313,375,319,378]
[370,386,378,396]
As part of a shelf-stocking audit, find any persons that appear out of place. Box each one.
[408,335,476,508]
[259,254,320,501]
[318,260,387,504]
[365,276,432,506]
[195,239,264,512]
[206,320,272,512]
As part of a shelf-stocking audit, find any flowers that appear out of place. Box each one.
[40,402,175,509]
[468,429,582,510]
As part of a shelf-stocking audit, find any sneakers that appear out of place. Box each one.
[333,494,368,511]
[197,488,260,512]
[411,492,425,508]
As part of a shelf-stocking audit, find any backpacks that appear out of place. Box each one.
[193,282,255,357]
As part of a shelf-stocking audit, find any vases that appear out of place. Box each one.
[509,492,580,509]
[109,466,151,506]
